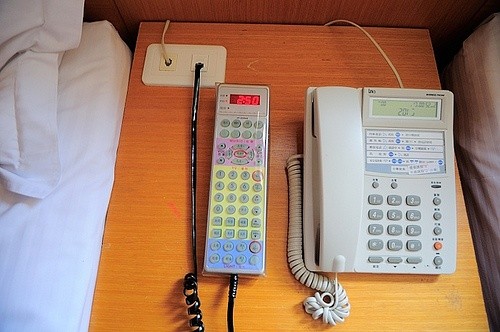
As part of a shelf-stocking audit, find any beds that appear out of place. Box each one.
[0,0,134,332]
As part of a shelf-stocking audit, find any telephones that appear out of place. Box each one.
[303,87,457,274]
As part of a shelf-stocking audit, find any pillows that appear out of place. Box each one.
[0,50,65,198]
[0,0,85,71]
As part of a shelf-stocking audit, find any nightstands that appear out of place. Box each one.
[88,21,491,332]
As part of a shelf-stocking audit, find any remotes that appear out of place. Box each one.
[205,83,269,276]
